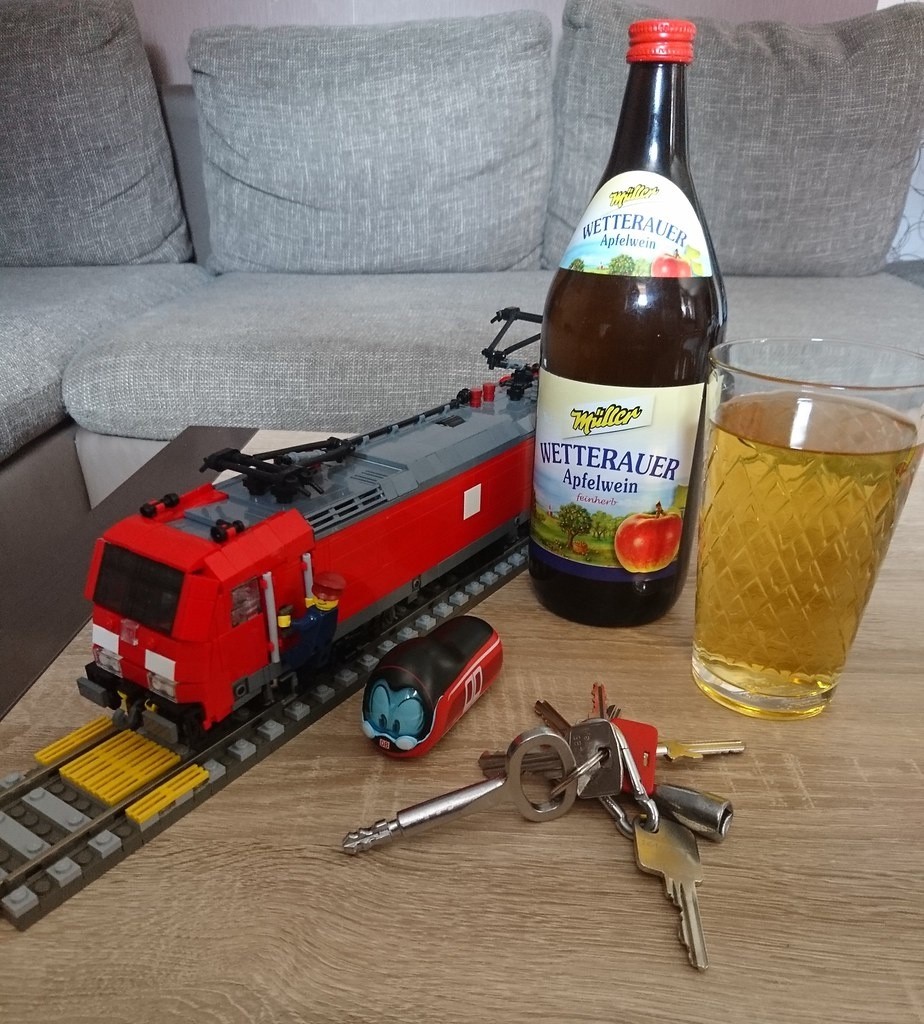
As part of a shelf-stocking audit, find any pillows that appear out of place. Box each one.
[192,16,555,272]
[1,0,197,268]
[546,1,924,276]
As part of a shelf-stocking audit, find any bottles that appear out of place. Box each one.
[527,20,728,628]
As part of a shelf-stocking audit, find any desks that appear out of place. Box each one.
[1,432,924,1024]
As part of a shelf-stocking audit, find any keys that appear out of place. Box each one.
[338,680,747,970]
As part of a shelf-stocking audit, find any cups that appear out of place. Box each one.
[693,337,924,720]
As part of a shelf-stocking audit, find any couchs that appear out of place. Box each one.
[0,85,924,692]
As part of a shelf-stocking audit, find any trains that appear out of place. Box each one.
[74,308,545,740]
